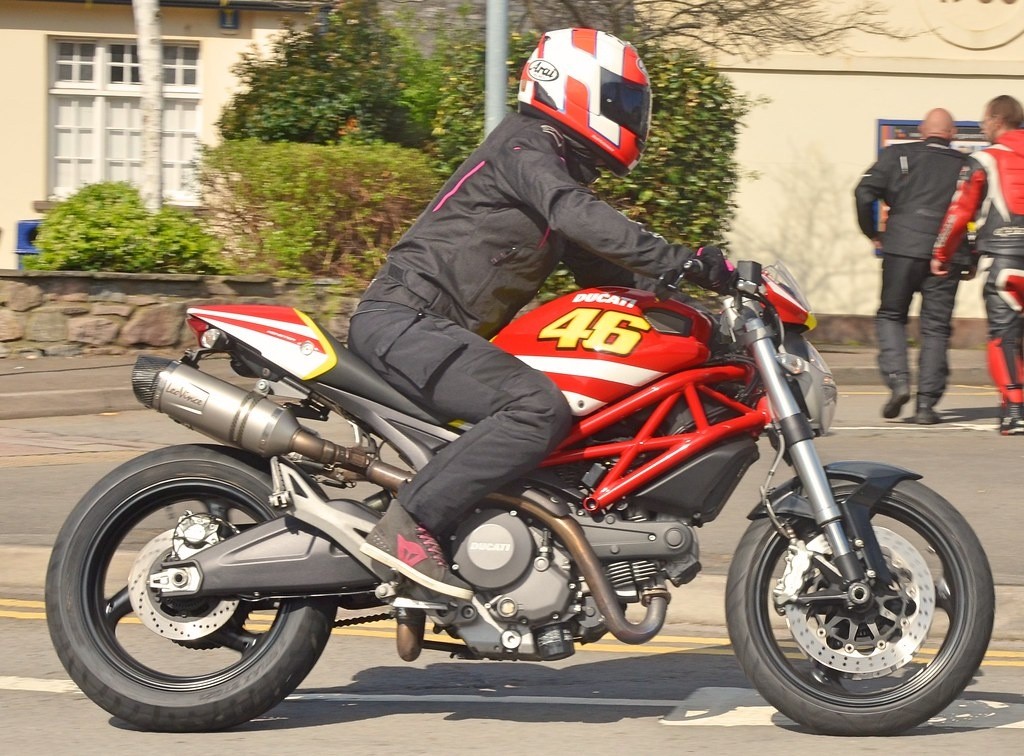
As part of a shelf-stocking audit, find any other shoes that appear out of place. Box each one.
[997,402,1024,436]
[915,395,942,423]
[884,383,910,419]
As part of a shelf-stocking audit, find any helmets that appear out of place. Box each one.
[516,26,653,175]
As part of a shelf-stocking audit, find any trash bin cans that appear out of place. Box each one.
[13,219,46,270]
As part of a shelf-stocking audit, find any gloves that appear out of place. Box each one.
[685,245,735,298]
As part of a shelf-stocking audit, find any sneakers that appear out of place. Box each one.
[360,498,475,602]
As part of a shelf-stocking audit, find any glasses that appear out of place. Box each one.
[977,117,994,129]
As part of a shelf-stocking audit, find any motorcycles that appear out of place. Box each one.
[40,250,999,738]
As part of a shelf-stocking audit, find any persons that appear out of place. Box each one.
[854,107,979,424]
[928,94,1024,434]
[346,28,738,600]
